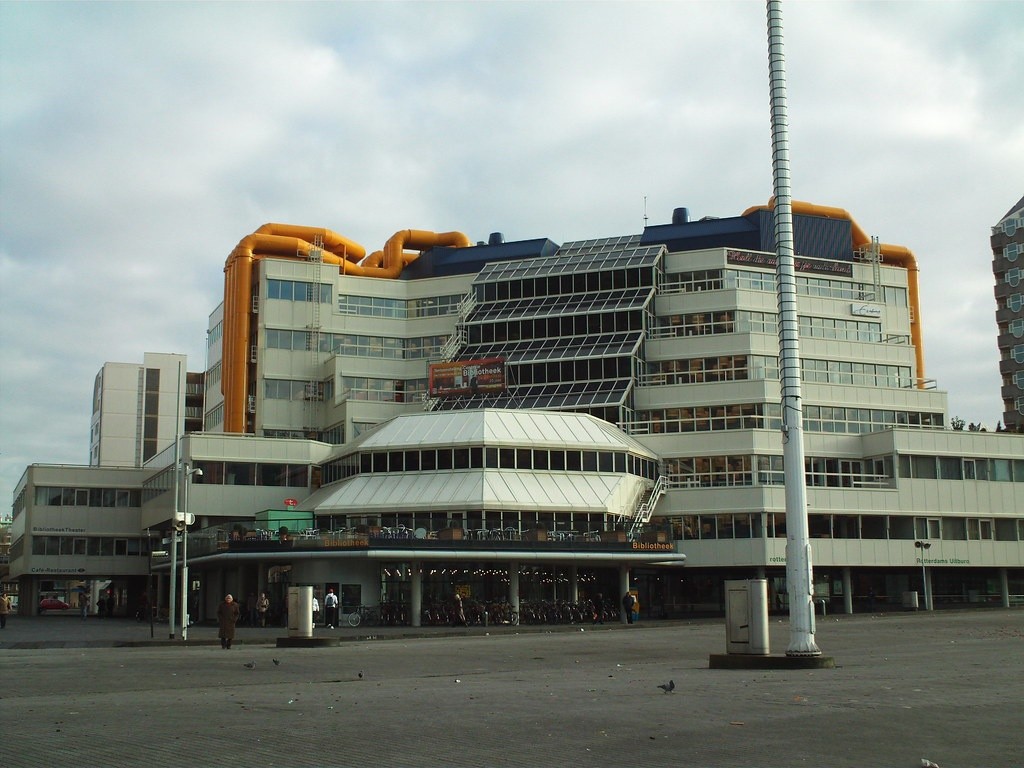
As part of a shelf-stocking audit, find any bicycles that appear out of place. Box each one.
[136,608,144,622]
[347,595,623,629]
[78,602,87,620]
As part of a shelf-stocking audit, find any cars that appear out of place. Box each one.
[40,598,69,612]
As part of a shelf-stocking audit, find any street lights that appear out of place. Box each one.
[181,464,204,641]
[915,540,931,611]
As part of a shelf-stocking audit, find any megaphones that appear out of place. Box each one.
[174,519,186,531]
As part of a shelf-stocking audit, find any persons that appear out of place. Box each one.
[0,594,12,628]
[623,592,634,624]
[452,592,469,627]
[218,593,239,650]
[96,596,105,618]
[80,594,89,623]
[256,592,270,628]
[595,592,605,625]
[107,595,114,618]
[313,594,320,628]
[324,589,339,630]
[775,595,781,610]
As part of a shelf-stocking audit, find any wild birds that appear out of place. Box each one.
[272,655,281,667]
[358,670,364,680]
[657,680,676,695]
[241,660,256,672]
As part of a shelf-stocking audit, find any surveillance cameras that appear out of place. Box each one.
[196,467,203,476]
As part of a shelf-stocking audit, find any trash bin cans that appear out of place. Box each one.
[286,585,315,637]
[902,591,919,612]
[723,579,770,654]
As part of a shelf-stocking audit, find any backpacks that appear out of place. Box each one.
[326,594,333,606]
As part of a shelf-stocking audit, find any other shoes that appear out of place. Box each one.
[312,623,315,628]
[222,644,225,648]
[227,646,230,649]
[331,626,334,630]
[327,624,331,628]
